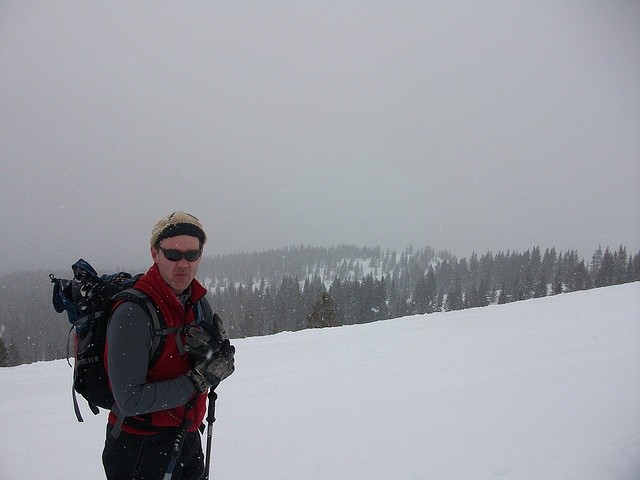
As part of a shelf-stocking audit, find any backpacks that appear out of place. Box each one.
[48,257,205,422]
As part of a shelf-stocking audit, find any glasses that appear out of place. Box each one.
[158,246,203,262]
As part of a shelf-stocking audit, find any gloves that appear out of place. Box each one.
[186,339,236,395]
[184,313,227,363]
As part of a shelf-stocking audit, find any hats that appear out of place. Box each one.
[150,212,207,248]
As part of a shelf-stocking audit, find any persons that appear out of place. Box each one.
[99,210,235,480]
